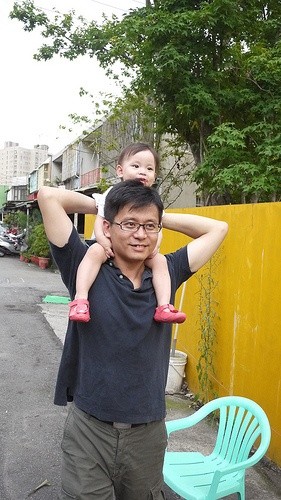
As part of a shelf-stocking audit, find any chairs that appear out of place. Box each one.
[162,395,272,500]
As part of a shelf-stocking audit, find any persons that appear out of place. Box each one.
[69,143,186,324]
[38,184,230,500]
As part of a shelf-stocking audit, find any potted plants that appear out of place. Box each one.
[20,223,50,270]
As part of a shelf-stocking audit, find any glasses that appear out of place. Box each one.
[111,221,161,232]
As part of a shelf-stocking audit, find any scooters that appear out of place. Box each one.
[0,220,29,258]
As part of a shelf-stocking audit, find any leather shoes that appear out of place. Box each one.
[68,300,89,323]
[152,305,185,324]
